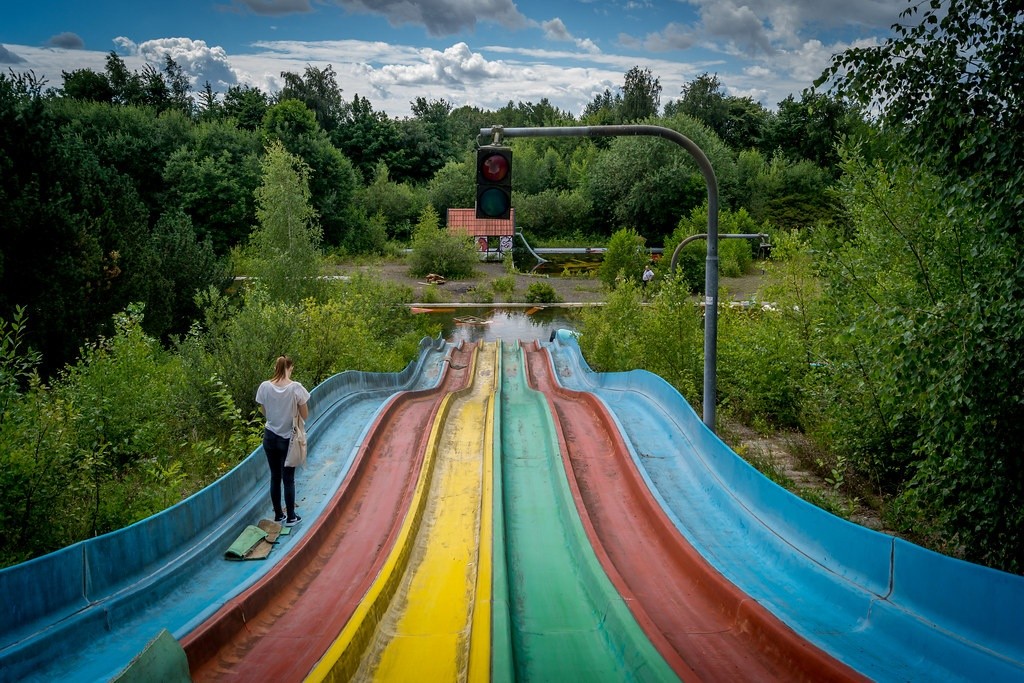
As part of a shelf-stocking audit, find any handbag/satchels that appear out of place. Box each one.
[285,414,306,466]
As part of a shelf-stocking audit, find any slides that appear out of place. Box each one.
[0,328,1024,682]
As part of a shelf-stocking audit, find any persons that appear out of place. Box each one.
[642,264,654,290]
[255,354,312,527]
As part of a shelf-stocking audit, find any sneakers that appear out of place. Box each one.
[284,513,302,526]
[273,515,287,524]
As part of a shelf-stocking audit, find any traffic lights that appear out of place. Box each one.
[475,145,513,220]
